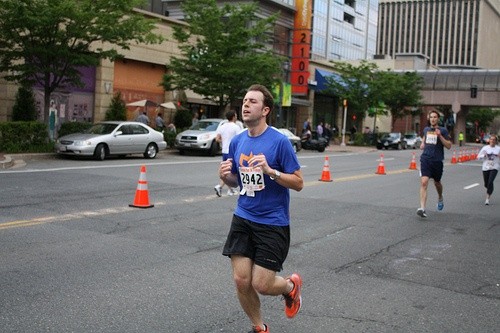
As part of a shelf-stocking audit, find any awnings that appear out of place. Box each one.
[314,68,369,95]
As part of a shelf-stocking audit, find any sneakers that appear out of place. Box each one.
[253,323,269,333]
[282,273,302,318]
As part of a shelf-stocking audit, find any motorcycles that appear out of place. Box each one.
[300,131,329,152]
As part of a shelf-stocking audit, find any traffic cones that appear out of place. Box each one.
[128,165,156,209]
[374,152,387,175]
[452,148,480,164]
[318,155,334,183]
[408,150,417,170]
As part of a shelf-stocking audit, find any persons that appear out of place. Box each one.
[137,112,149,124]
[477,134,500,204]
[201,112,207,119]
[219,84,304,333]
[351,125,356,141]
[459,131,463,146]
[168,121,176,133]
[365,127,370,133]
[157,113,164,129]
[416,110,451,218]
[317,122,323,139]
[323,123,332,146]
[304,117,309,130]
[192,113,199,125]
[302,125,312,140]
[214,111,242,197]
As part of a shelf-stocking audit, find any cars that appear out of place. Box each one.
[56,121,168,160]
[377,133,408,149]
[403,133,423,148]
[277,128,302,153]
[173,119,246,157]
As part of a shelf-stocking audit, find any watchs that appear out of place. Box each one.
[270,170,280,180]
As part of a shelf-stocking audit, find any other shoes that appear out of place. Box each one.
[485,199,489,205]
[228,188,238,195]
[417,208,427,217]
[438,196,443,210]
[214,185,222,197]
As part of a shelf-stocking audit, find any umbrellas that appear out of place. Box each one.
[160,101,188,120]
[127,99,160,111]
